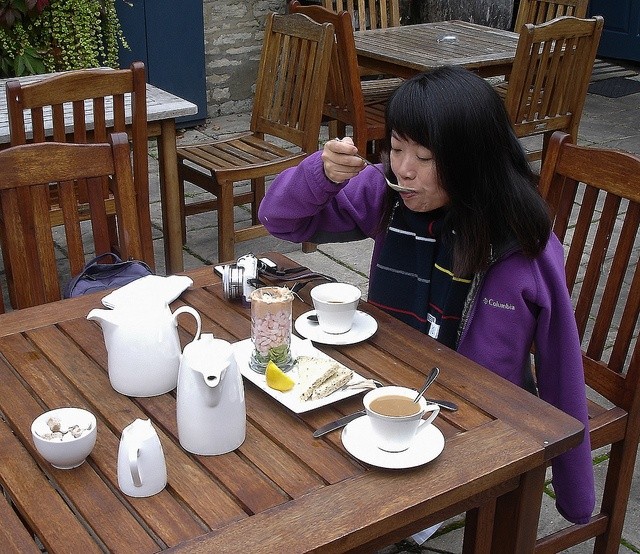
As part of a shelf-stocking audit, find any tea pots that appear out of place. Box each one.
[85,284,203,397]
[177,332,247,456]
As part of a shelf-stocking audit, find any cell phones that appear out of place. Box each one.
[214,257,277,277]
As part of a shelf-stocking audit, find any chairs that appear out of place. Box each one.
[1,132,153,313]
[7,62,156,312]
[511,1,590,42]
[285,0,384,163]
[485,16,606,160]
[175,11,335,263]
[464,131,640,548]
[318,0,402,30]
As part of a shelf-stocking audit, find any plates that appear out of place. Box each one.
[294,309,379,345]
[230,332,371,415]
[340,416,445,470]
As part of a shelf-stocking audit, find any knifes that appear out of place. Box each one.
[313,409,367,439]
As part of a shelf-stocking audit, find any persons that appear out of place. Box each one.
[257,65,596,548]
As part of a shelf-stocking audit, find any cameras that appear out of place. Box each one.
[221,251,259,305]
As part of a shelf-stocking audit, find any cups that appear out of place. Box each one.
[310,282,362,334]
[363,384,440,453]
[117,418,167,498]
[249,286,294,375]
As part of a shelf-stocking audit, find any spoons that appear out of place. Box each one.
[333,136,415,193]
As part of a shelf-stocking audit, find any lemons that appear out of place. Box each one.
[265,360,294,391]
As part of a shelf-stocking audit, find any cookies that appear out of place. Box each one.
[313,364,353,400]
[293,351,338,402]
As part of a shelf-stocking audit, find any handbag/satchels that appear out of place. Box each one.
[64,252,154,299]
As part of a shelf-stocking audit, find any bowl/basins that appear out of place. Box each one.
[30,407,97,470]
